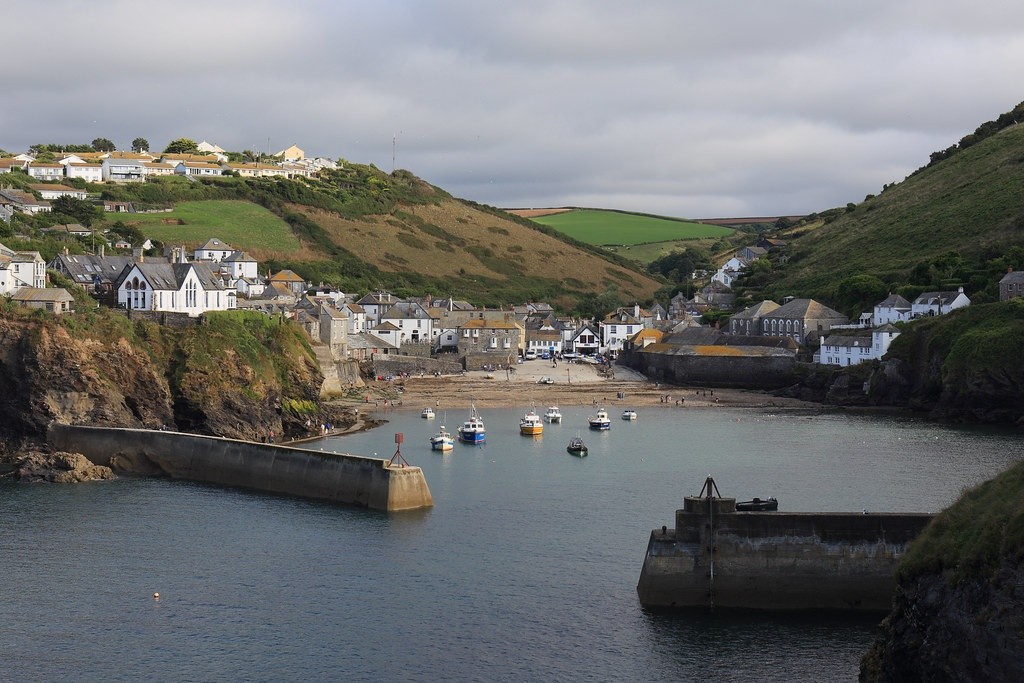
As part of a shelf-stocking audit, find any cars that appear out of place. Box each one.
[542,353,550,359]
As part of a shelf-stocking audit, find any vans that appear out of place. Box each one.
[526,349,537,360]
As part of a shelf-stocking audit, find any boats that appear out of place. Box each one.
[544,397,562,423]
[736,494,778,513]
[421,407,435,419]
[429,409,455,451]
[567,427,588,457]
[519,400,543,435]
[587,407,610,431]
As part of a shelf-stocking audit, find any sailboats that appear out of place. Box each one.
[457,391,486,445]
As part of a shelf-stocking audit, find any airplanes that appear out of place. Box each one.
[621,409,637,420]
